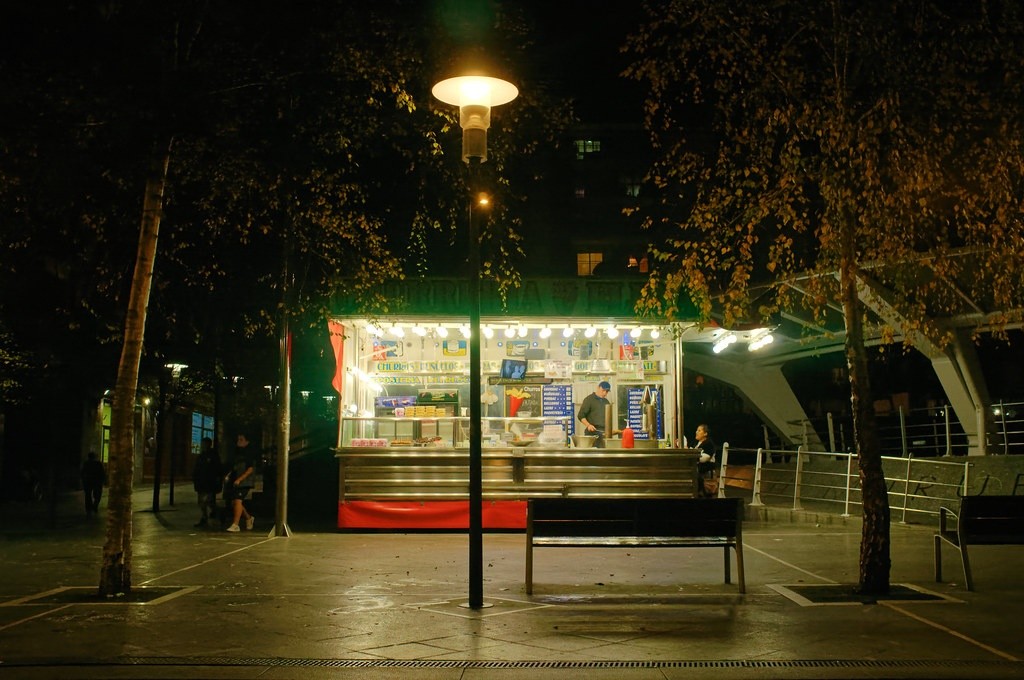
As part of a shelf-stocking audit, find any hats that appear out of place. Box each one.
[598,381,611,391]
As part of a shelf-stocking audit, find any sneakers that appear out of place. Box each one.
[246,516,255,530]
[226,523,241,532]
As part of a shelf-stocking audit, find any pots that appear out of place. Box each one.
[568,435,599,448]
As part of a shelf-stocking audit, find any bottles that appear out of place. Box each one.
[666,433,671,447]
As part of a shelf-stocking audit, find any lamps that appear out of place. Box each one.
[747,332,773,352]
[713,332,737,353]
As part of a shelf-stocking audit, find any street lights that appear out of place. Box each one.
[163,357,189,506]
[432,46,520,608]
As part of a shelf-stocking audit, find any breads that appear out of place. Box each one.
[390,436,444,445]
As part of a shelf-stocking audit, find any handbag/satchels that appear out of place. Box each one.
[238,472,255,487]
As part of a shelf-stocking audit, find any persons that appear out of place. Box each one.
[192,437,225,527]
[501,361,526,380]
[223,433,255,532]
[79,452,107,517]
[578,382,623,448]
[684,425,717,497]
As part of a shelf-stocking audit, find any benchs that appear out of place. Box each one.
[525,496,746,597]
[934,495,1024,591]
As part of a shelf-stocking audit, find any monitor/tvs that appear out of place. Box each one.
[500,359,528,381]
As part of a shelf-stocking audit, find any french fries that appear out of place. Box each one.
[572,338,590,356]
[506,387,531,399]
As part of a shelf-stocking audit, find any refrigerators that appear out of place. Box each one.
[375,396,417,442]
[416,388,460,447]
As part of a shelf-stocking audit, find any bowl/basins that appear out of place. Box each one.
[517,411,531,418]
[507,441,535,447]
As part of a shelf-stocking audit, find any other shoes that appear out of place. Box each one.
[194,518,208,528]
